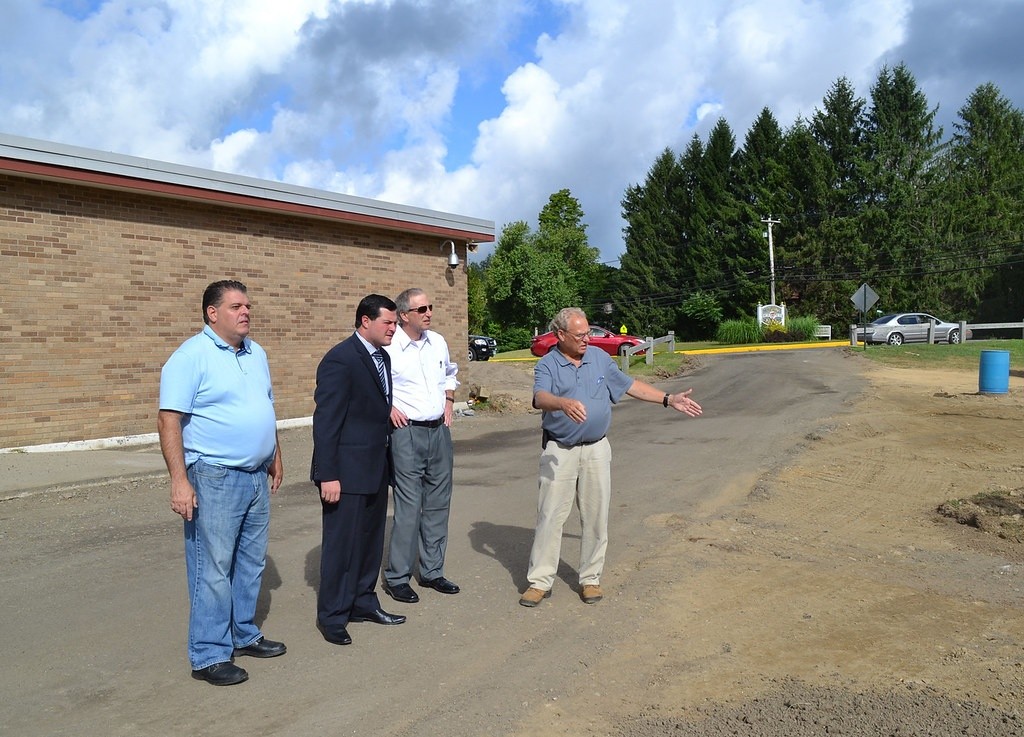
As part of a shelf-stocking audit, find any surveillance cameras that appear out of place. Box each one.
[448,254,460,269]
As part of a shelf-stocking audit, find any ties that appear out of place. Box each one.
[373,350,387,396]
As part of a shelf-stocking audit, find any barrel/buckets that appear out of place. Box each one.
[979,349,1010,394]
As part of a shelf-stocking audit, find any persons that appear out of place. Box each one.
[520,306,701,605]
[309,292,407,646]
[382,287,460,604]
[157,280,289,686]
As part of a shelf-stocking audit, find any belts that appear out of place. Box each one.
[401,413,445,428]
[577,434,606,447]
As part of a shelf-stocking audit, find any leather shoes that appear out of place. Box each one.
[579,584,603,604]
[316,624,352,645]
[349,608,406,625]
[192,662,248,685]
[519,585,552,607]
[419,576,460,593]
[233,636,287,658]
[385,583,419,602]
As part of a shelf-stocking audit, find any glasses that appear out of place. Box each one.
[561,327,593,342]
[409,304,433,313]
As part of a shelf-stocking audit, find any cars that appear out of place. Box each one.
[856,311,961,345]
[529,324,647,357]
[467,334,498,361]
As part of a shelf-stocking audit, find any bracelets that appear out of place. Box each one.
[663,393,671,408]
[446,396,455,403]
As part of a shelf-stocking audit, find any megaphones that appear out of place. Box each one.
[468,241,479,253]
